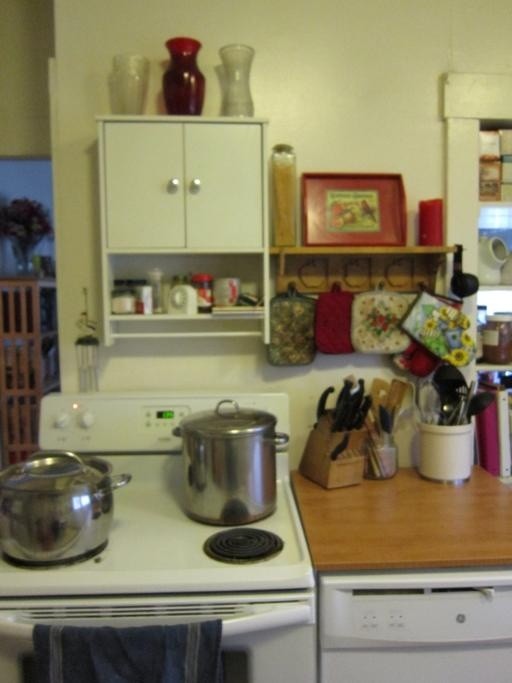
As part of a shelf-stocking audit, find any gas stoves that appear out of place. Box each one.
[2,454,316,591]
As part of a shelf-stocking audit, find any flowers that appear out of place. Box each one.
[0,197,54,269]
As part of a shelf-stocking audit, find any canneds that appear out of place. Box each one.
[192,274,213,313]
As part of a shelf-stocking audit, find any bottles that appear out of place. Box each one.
[272,144,296,247]
[110,36,254,116]
[476,305,488,363]
[113,266,168,314]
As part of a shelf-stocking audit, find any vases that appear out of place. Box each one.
[13,246,36,279]
[111,37,255,118]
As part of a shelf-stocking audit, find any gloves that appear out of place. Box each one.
[396,290,478,369]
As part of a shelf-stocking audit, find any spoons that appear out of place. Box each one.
[438,396,460,426]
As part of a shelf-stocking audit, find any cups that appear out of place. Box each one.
[212,275,240,306]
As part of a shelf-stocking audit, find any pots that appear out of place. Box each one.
[0,447,133,567]
[175,401,288,526]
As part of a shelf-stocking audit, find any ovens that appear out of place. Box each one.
[2,593,319,683]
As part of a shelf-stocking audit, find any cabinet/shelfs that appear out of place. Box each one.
[0,279,62,466]
[95,113,270,347]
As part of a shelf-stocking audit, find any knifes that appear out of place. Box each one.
[317,380,372,460]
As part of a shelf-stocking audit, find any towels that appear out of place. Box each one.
[31,621,225,683]
